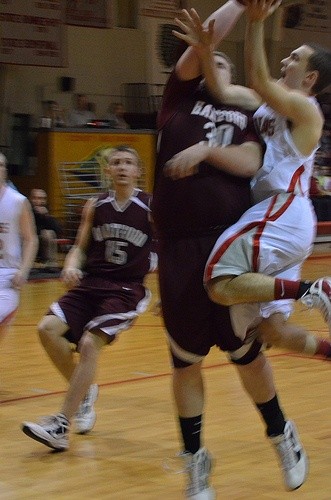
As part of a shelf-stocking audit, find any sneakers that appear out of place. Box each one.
[177,447,216,500]
[264,420,309,493]
[73,383,98,434]
[300,276,331,331]
[21,412,71,451]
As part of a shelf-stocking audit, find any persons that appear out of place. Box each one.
[21,145,159,451]
[171,0,331,362]
[44,93,129,130]
[156,0,306,500]
[0,152,62,334]
